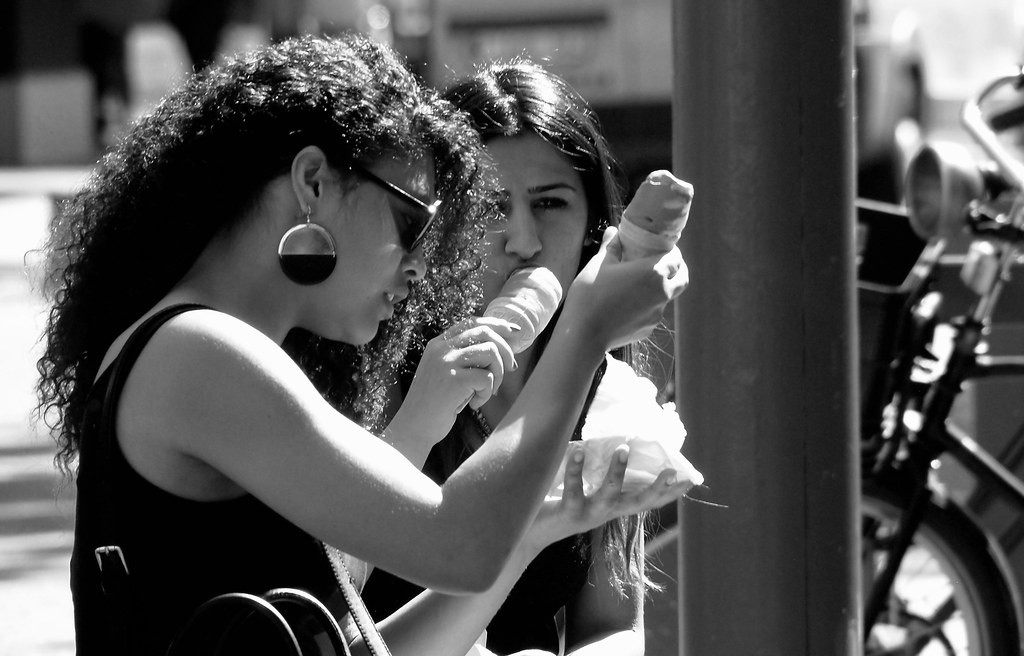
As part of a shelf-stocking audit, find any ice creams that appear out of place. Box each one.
[619,168,695,267]
[475,264,565,352]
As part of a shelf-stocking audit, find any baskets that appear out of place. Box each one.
[851,196,949,441]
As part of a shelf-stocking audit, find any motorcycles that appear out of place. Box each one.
[860,71,1024,655]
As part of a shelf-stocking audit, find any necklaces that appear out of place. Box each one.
[468,401,593,567]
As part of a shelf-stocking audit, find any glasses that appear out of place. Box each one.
[348,164,444,253]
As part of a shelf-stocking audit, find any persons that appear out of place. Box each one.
[342,61,656,656]
[23,34,696,656]
